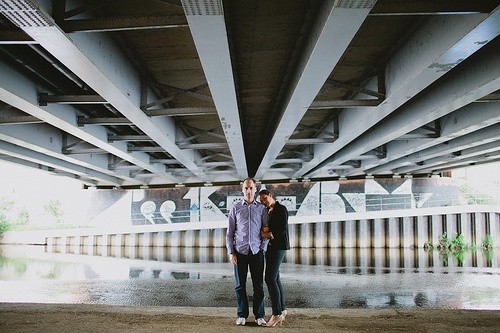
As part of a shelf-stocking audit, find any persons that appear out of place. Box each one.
[255,189,290,328]
[227,177,270,327]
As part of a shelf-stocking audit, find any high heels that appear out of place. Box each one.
[265,310,287,327]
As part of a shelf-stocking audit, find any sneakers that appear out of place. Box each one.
[236,317,246,326]
[254,317,266,326]
